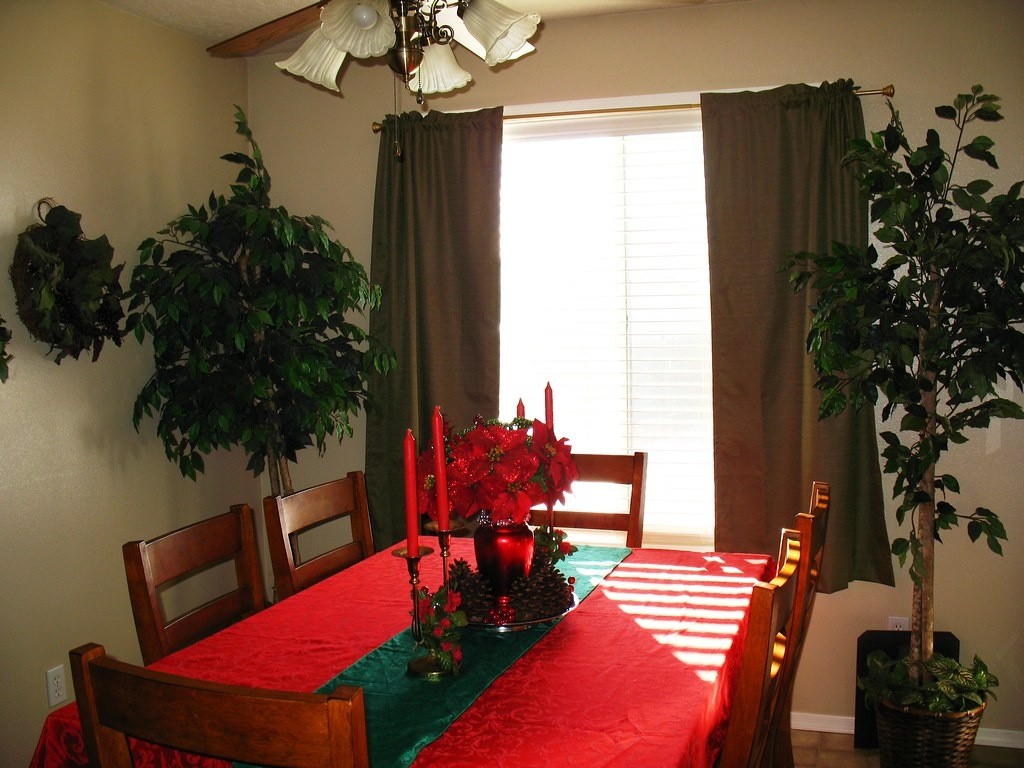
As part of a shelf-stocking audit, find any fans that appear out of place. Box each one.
[204,0,535,63]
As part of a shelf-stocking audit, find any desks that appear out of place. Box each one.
[28,534,776,767]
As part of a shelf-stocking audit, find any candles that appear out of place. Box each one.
[516,397,525,418]
[545,381,554,429]
[432,404,451,531]
[402,427,419,557]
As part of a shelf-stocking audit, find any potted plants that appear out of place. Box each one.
[775,84,1023,768]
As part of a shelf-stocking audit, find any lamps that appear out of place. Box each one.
[272,0,540,155]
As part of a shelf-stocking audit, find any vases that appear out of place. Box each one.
[472,511,534,595]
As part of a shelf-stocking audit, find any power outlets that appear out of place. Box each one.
[46,664,67,707]
[888,616,909,630]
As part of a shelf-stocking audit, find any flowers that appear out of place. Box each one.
[410,581,467,678]
[412,413,579,527]
[531,522,579,567]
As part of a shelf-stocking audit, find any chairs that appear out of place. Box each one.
[776,479,831,767]
[69,642,369,767]
[528,452,648,547]
[263,470,376,601]
[719,527,801,768]
[122,502,266,666]
